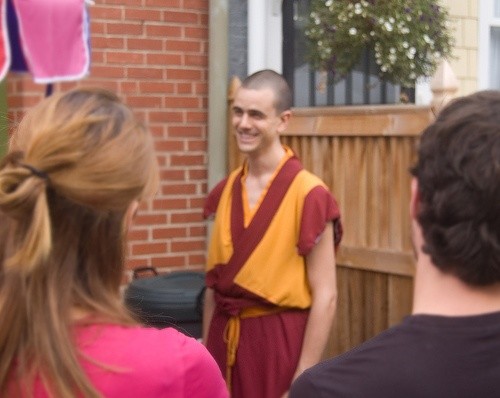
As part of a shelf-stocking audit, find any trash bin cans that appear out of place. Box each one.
[121,267,208,340]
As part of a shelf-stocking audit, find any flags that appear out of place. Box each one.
[0,0,95,87]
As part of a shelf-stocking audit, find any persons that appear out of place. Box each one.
[0,87,235,398]
[287,89,500,398]
[203,68,343,398]
